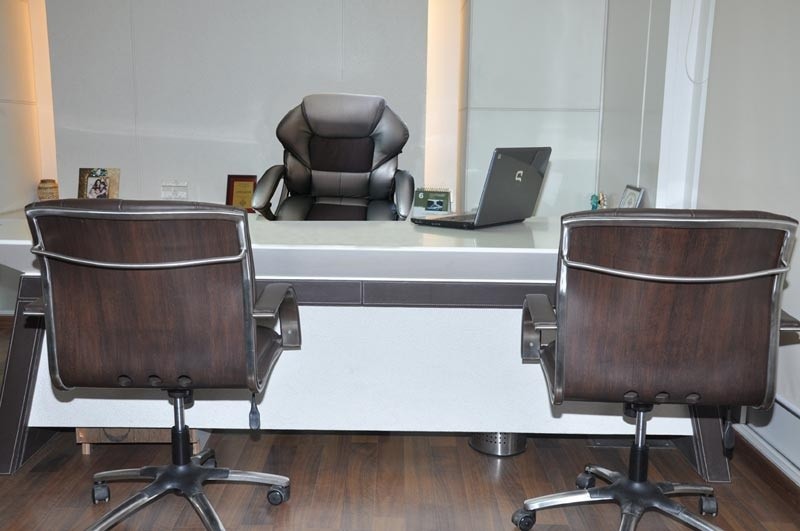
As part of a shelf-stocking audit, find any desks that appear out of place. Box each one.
[0,216,695,456]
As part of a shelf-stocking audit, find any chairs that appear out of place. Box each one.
[511,210,800,531]
[253,92,415,225]
[24,198,302,531]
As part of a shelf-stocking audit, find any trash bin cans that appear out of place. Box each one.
[468,431,528,458]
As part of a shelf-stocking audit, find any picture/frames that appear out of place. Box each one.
[77,167,121,199]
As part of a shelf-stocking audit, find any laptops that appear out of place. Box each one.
[411,147,552,230]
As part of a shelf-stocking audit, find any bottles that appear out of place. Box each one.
[37,179,59,201]
